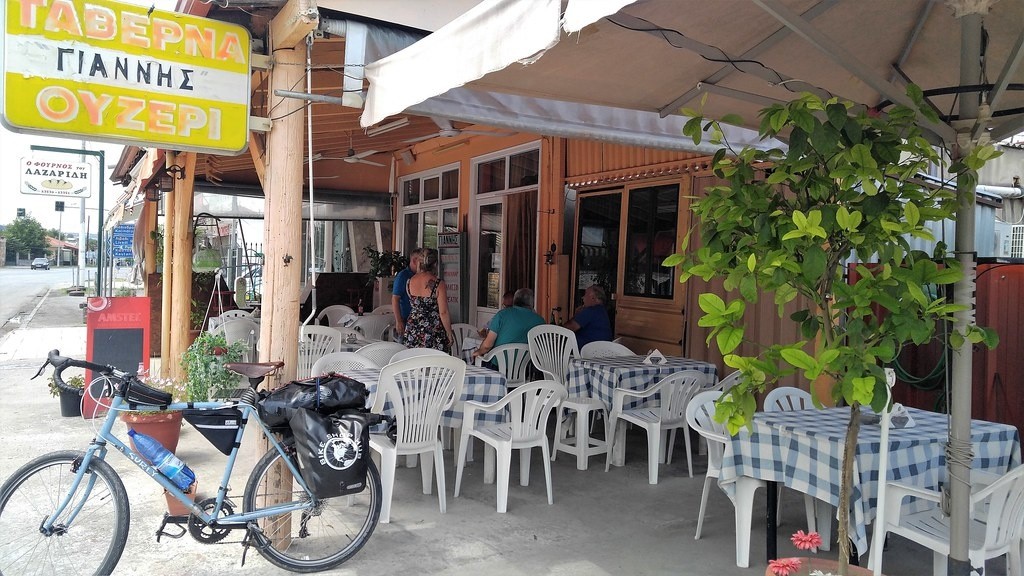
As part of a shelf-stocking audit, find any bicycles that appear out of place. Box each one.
[0,348,383,576]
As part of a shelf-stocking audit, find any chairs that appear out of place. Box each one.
[208,303,1024,576]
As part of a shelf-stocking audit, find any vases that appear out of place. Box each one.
[119,414,184,465]
[164,481,198,515]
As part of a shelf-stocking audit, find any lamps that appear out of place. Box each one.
[303,153,323,165]
[366,117,410,137]
[157,173,174,192]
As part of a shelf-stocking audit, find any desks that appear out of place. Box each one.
[461,335,492,366]
[331,360,509,485]
[301,338,376,353]
[560,353,718,466]
[717,403,1022,574]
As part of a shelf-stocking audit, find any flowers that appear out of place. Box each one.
[96,364,193,415]
[768,529,822,576]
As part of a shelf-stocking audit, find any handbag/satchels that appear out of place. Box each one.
[288,407,369,499]
[259,373,368,426]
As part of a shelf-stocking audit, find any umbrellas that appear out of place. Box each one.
[361,0,1024,576]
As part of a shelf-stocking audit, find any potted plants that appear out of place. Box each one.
[179,329,248,402]
[47,374,84,416]
[659,80,1005,576]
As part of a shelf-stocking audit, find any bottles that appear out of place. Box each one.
[358,299,364,316]
[127,428,196,491]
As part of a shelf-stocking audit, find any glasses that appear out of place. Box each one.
[502,303,513,308]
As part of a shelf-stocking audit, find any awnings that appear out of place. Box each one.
[103,189,202,231]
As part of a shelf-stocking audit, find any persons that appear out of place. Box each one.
[472,287,546,380]
[392,248,421,344]
[562,284,612,355]
[404,247,454,353]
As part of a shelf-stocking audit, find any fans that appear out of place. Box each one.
[318,131,387,167]
[400,116,519,143]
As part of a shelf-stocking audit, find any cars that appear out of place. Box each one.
[31,258,49,270]
